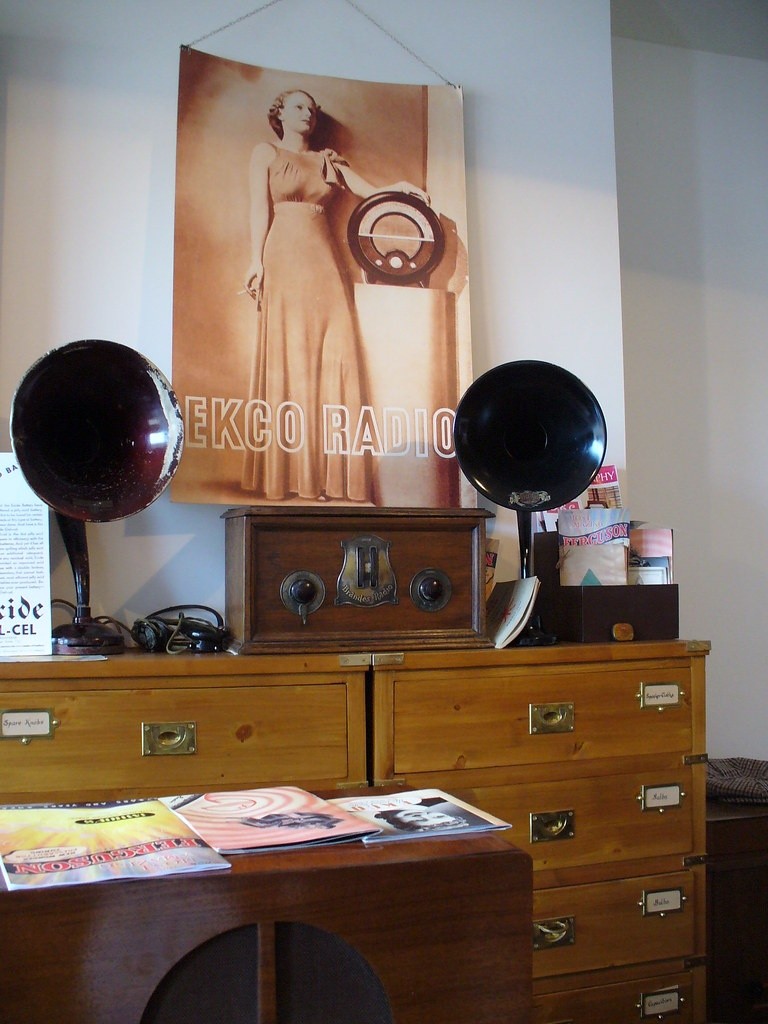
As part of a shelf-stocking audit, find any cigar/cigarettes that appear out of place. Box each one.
[237,287,255,295]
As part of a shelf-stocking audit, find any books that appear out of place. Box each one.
[156,786,385,855]
[326,788,512,844]
[486,576,541,649]
[558,509,674,586]
[543,466,624,531]
[0,797,232,892]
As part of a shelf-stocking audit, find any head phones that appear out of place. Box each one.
[131,604,228,652]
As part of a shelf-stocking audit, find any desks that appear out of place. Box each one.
[706,792,768,1024]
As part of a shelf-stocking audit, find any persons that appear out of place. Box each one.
[348,798,470,832]
[237,89,431,500]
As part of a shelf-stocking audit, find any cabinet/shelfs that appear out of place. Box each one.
[0,652,372,805]
[0,781,535,1024]
[370,640,711,1024]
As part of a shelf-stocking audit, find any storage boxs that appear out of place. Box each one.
[514,584,680,648]
[218,505,498,655]
